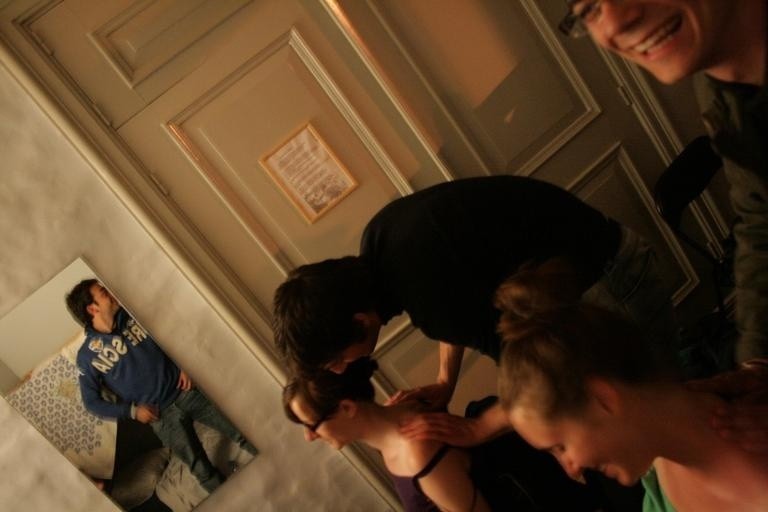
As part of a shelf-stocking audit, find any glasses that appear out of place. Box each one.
[558,0,615,39]
[306,416,329,432]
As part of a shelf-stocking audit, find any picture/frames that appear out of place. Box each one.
[259,121,357,226]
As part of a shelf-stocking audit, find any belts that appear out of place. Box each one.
[580,217,622,297]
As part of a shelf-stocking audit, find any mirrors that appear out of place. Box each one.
[0,256,259,512]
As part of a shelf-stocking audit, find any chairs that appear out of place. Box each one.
[467,395,619,511]
[651,134,742,315]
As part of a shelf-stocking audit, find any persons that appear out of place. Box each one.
[559,0,768,370]
[270,174,672,512]
[494,255,768,512]
[282,358,493,512]
[67,279,258,495]
